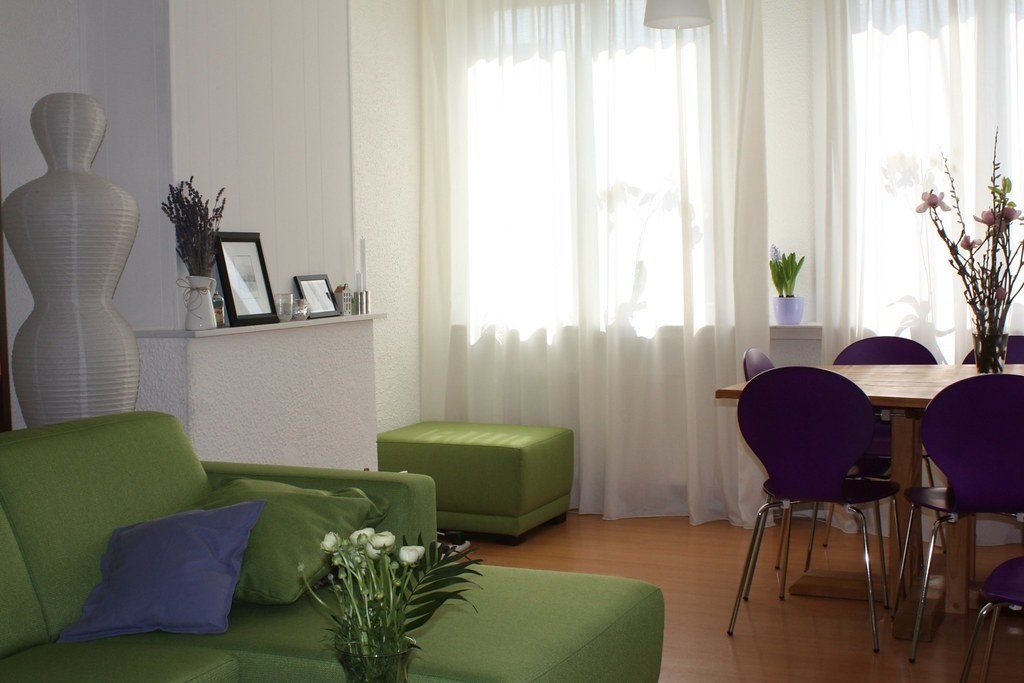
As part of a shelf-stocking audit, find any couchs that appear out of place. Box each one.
[0,411,664,683]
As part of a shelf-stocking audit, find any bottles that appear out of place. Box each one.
[211,290,225,328]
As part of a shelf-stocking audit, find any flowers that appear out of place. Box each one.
[296,526,486,683]
[769,246,805,299]
[915,126,1024,374]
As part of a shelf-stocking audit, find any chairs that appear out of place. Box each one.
[727,336,1024,683]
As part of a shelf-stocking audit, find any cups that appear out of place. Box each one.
[273,294,293,322]
[293,298,311,321]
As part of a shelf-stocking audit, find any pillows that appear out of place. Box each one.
[183,479,391,607]
[57,501,266,642]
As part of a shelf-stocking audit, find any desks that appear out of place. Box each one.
[716,364,1024,642]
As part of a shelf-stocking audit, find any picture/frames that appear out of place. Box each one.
[210,231,280,327]
[293,274,341,319]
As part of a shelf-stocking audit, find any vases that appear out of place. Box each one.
[771,296,804,325]
[336,636,416,683]
[184,276,218,330]
[969,305,1011,372]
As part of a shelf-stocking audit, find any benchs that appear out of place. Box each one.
[377,422,574,547]
